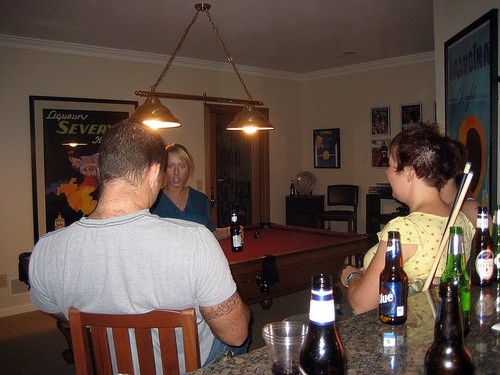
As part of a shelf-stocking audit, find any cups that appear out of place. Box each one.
[262,320,308,375]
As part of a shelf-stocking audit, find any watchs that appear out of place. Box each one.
[345,271,361,286]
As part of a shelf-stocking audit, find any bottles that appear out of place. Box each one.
[379,327,407,375]
[473,286,496,365]
[471,206,494,287]
[298,273,348,375]
[492,205,500,286]
[423,280,476,375]
[378,231,408,326]
[441,226,472,337]
[290,179,295,196]
[230,208,243,252]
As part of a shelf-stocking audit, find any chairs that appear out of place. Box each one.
[67,307,201,375]
[316,185,359,264]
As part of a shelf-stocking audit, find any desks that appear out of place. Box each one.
[217,221,371,308]
[181,262,500,375]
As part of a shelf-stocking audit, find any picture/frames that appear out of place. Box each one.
[29,96,138,247]
[445,7,499,216]
[369,105,391,136]
[371,138,391,168]
[313,128,340,169]
[400,103,422,132]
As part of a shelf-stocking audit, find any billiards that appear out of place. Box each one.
[254,232,260,237]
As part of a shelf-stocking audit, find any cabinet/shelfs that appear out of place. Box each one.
[366,193,409,235]
[286,194,324,228]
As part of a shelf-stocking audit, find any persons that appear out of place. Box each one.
[28,118,250,375]
[371,107,417,167]
[340,119,495,315]
[149,143,245,245]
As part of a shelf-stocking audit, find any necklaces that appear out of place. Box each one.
[165,188,184,207]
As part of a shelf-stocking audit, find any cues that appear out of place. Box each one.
[421,160,474,291]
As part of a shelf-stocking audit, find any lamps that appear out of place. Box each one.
[135,3,275,134]
